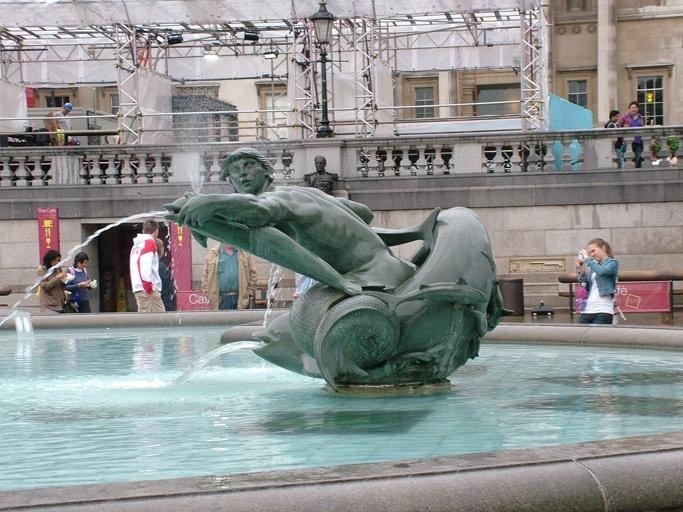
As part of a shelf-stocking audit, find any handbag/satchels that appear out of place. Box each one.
[62,301,77,313]
[574,280,589,311]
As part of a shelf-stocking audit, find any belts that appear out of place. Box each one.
[219,291,238,296]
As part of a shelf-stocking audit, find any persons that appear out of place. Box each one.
[574,237,619,324]
[605,110,625,168]
[201,241,257,310]
[59,252,96,313]
[649,156,663,166]
[175,146,419,290]
[152,235,175,310]
[665,151,678,168]
[128,220,168,313]
[620,103,646,167]
[51,101,75,136]
[35,250,70,314]
[302,154,338,196]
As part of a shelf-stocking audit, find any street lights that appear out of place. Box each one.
[297,0,351,142]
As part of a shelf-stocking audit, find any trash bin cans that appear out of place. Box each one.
[498,279,524,316]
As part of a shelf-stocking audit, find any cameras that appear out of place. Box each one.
[577,251,585,261]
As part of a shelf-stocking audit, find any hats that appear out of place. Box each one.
[64,103,72,111]
